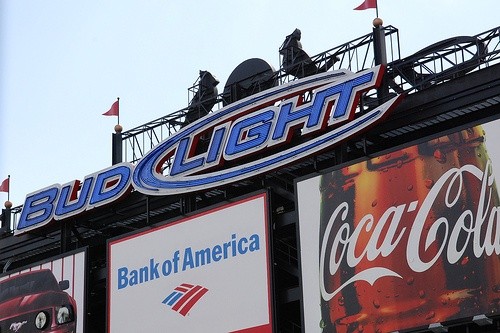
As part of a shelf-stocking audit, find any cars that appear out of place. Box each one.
[0,267,79,333]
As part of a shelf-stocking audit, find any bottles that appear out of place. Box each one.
[315,125,500,333]
